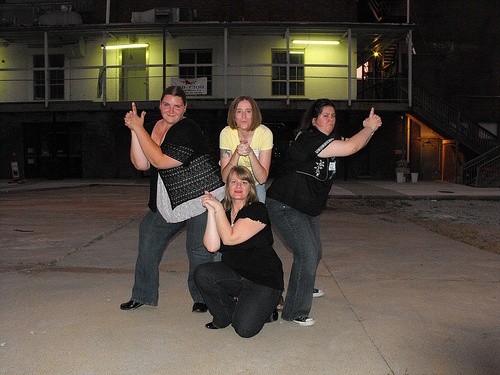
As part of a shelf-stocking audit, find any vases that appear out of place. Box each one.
[396,172,404,182]
[411,173,418,182]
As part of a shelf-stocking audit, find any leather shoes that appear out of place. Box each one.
[192,303,208,313]
[265,309,278,322]
[206,322,224,329]
[120,299,144,309]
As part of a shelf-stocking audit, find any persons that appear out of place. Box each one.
[120,86,227,313]
[219,96,285,311]
[193,165,284,338]
[266,98,382,326]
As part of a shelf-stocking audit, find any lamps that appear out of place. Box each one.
[101,43,149,50]
[291,39,341,45]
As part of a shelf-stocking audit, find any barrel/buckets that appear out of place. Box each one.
[397,173,404,182]
[411,173,418,182]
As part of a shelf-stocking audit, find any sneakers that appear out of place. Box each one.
[291,315,316,327]
[313,288,325,298]
